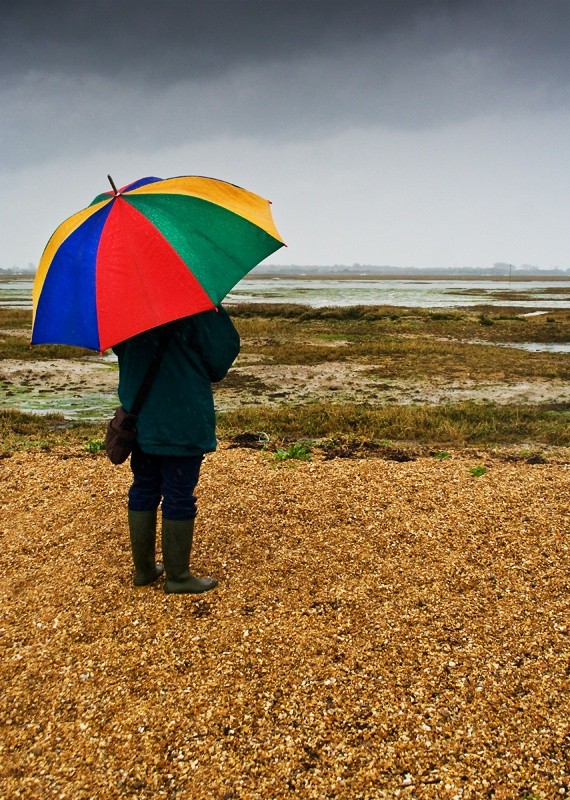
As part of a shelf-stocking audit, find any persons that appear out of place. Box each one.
[111,303,240,594]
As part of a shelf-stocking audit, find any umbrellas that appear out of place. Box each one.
[30,175,288,358]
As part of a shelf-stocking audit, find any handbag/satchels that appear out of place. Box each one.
[105,406,136,464]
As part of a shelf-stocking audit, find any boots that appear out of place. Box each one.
[128,511,165,587]
[161,519,218,593]
